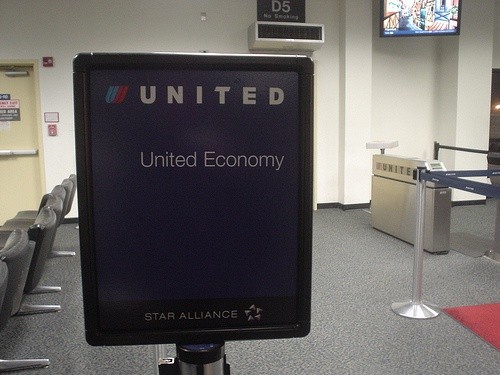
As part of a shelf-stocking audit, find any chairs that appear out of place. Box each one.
[0,175,76,371]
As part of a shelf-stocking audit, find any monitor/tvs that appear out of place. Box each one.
[380,0,462,37]
[72,52,313,345]
